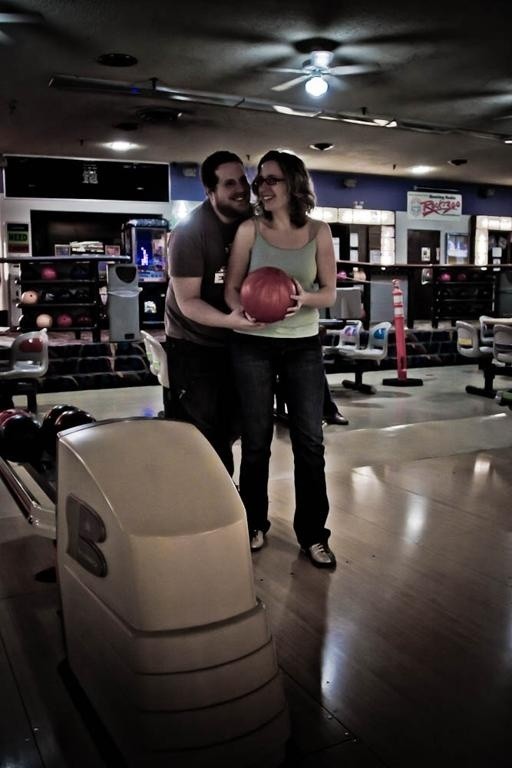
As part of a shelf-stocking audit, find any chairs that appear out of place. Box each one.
[454,314,512,407]
[388,326,457,370]
[140,331,169,418]
[323,318,400,395]
[48,343,155,393]
[1,331,50,412]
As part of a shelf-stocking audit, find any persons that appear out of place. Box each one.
[221,150,341,569]
[150,152,271,488]
[323,373,353,427]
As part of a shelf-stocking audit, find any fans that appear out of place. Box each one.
[258,51,382,93]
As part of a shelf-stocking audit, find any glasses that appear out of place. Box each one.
[254,173,287,186]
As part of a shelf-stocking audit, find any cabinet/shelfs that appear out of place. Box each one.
[427,268,501,329]
[14,263,102,343]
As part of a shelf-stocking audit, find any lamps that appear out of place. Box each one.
[303,76,329,97]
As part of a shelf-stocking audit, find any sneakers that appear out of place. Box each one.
[299,531,337,571]
[249,522,266,551]
[325,411,350,426]
